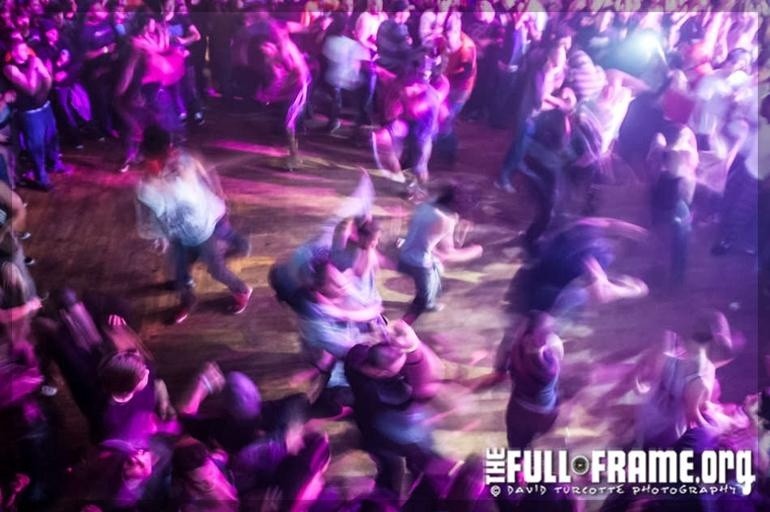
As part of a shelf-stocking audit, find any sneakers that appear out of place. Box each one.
[491,179,519,194]
[229,282,254,316]
[13,109,433,266]
[424,302,444,312]
[170,299,197,326]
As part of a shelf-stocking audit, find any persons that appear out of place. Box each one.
[0,0,770,509]
